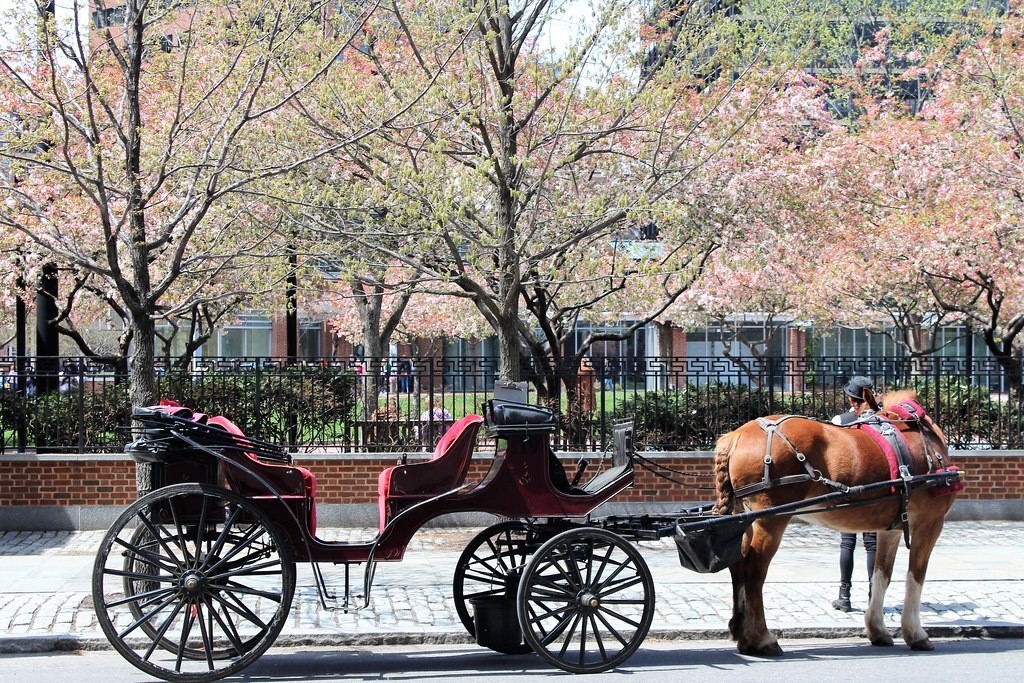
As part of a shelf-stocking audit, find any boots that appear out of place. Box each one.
[832,584,851,613]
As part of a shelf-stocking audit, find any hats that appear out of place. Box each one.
[843,375,876,400]
[401,355,409,361]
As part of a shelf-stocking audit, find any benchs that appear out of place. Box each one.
[379,414,486,535]
[345,420,456,454]
[205,415,316,534]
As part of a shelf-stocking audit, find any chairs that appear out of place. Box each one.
[481,397,571,494]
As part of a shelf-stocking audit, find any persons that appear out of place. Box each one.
[348,354,416,393]
[604,358,613,392]
[566,356,596,452]
[2,358,87,398]
[367,395,452,451]
[414,396,453,453]
[830,375,877,613]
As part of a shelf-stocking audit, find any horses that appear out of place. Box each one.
[710,388,959,658]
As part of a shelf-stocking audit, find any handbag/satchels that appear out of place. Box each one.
[400,371,408,376]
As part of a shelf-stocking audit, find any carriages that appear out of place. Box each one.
[90,380,967,683]
